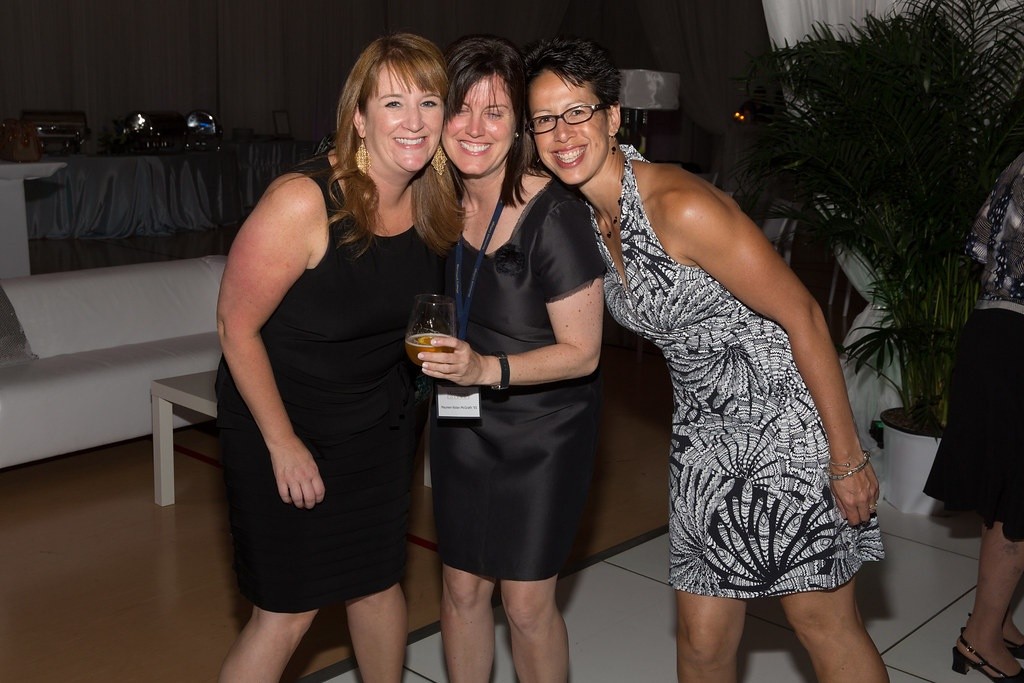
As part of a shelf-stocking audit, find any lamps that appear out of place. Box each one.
[617,69,682,157]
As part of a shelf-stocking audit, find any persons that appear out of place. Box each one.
[526,40,890,683]
[418,39,605,683]
[215,33,449,683]
[952,151,1024,683]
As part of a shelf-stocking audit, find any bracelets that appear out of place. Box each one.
[826,449,870,480]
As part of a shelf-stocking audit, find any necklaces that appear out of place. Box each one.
[606,178,624,238]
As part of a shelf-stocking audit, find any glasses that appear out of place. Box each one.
[525,104,610,135]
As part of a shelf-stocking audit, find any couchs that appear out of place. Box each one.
[0,257,224,471]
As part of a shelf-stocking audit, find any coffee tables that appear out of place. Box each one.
[151,367,218,507]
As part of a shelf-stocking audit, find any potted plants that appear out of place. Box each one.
[731,0,1023,515]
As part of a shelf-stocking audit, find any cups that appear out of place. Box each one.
[405,295,457,366]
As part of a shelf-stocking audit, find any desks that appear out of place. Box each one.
[25,135,315,244]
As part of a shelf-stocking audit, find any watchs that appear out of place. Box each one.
[491,351,510,390]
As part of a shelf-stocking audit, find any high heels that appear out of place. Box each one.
[952,627,1024,683]
[968,612,1024,659]
